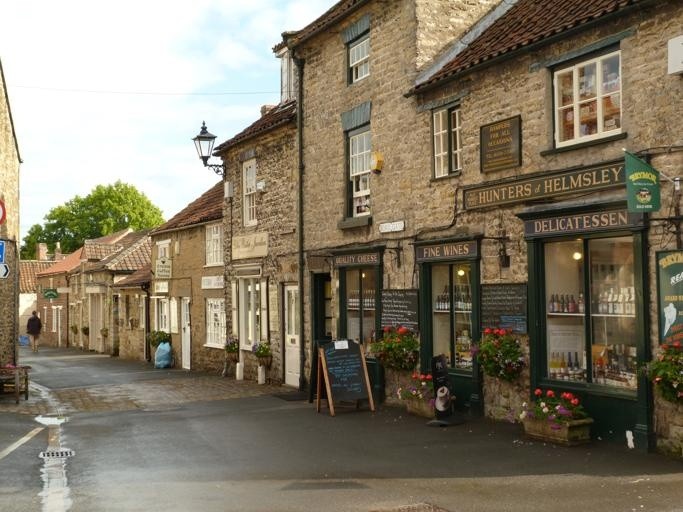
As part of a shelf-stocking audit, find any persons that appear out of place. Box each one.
[24,310,41,353]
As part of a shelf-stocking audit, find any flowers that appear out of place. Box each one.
[641,325,682,400]
[223,337,238,351]
[505,389,584,430]
[370,323,435,408]
[251,343,269,356]
[470,327,525,378]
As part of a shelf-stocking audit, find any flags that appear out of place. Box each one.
[623,151,661,215]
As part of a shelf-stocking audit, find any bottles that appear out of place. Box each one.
[435,285,471,310]
[442,330,472,369]
[349,289,375,308]
[550,342,637,388]
[549,287,635,315]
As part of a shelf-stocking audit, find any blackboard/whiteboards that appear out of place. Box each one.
[480,283,528,334]
[319,339,372,401]
[380,289,419,332]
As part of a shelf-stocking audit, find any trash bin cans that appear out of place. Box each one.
[361,355,384,409]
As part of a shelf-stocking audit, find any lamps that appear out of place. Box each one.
[191,121,226,181]
